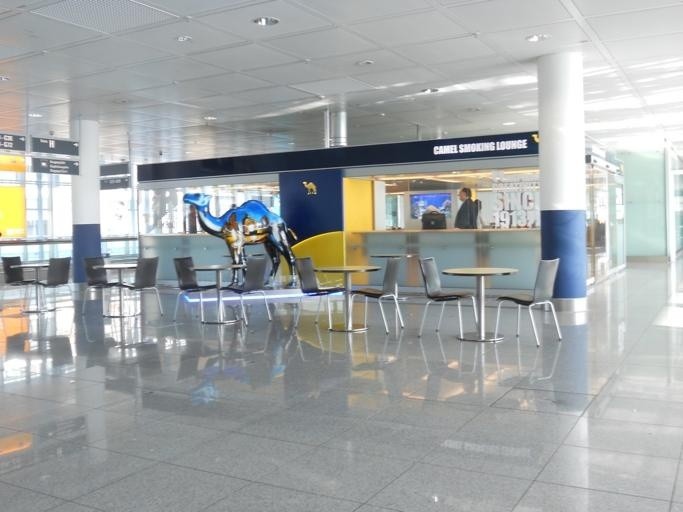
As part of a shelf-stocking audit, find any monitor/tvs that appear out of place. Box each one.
[422,214,446,229]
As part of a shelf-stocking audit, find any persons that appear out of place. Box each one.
[454,188,490,229]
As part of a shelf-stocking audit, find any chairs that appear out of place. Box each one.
[494,258,562,347]
[418,257,478,338]
[120,257,164,317]
[219,256,271,326]
[32,257,73,310]
[294,257,347,330]
[82,257,121,317]
[174,257,226,323]
[350,258,405,334]
[1,256,36,312]
